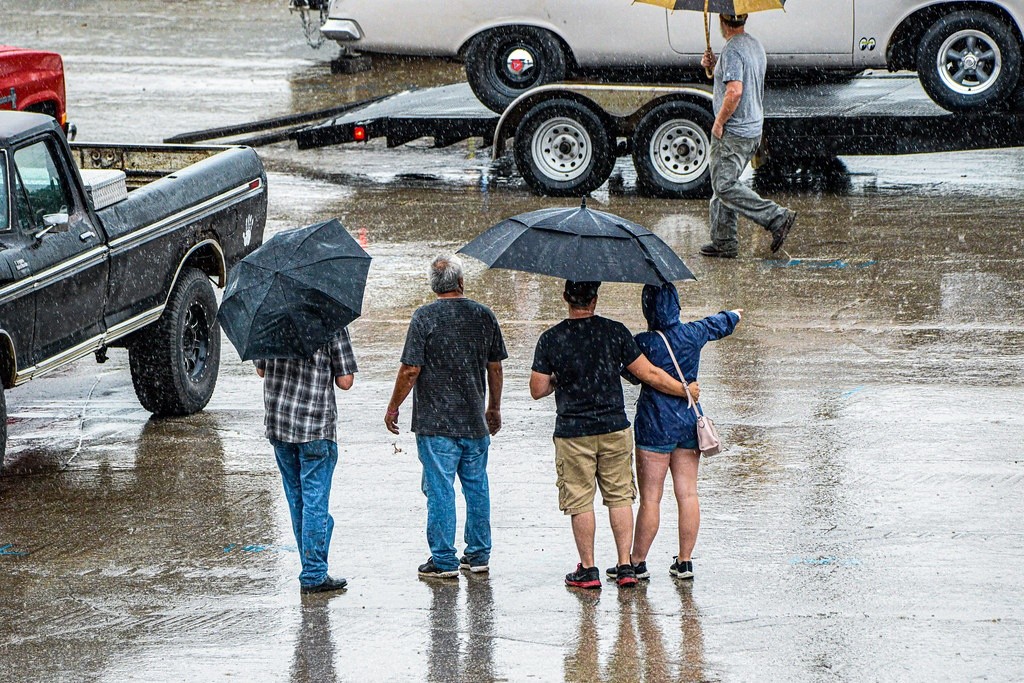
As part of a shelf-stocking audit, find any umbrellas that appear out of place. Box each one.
[631,0,785,79]
[455,195,697,289]
[216,218,372,363]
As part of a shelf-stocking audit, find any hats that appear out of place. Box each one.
[720,13,748,21]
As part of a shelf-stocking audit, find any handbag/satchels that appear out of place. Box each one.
[696,415,722,458]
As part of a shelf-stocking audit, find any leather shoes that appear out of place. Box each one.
[300,575,348,594]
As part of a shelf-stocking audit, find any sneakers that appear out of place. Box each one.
[770,210,797,254]
[669,556,694,580]
[606,554,650,580]
[565,563,602,589]
[616,564,639,587]
[699,243,738,258]
[417,555,459,578]
[459,556,489,573]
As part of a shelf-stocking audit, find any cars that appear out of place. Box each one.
[288,0,1024,113]
[0,42,70,139]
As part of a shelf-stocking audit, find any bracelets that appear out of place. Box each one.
[388,411,400,415]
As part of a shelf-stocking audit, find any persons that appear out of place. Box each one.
[619,282,743,579]
[384,252,509,577]
[530,279,700,588]
[253,326,359,593]
[699,13,797,257]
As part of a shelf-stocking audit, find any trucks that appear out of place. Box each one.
[292,69,1024,196]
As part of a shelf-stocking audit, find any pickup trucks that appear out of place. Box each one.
[0,108,267,474]
